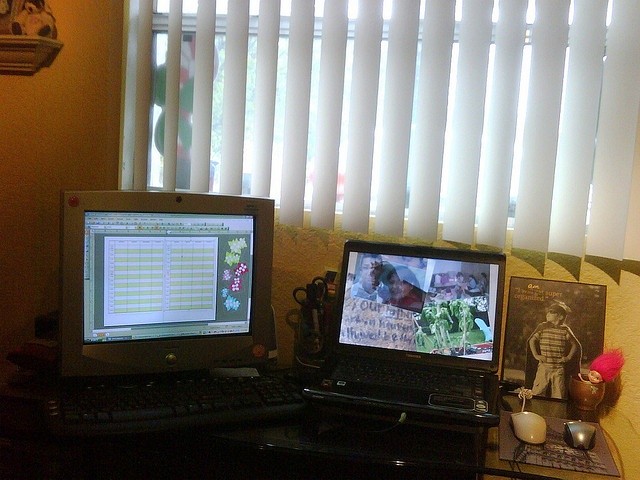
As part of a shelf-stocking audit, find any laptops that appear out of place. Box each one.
[302,239,505,428]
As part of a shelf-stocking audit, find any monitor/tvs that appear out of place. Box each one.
[62,191,275,375]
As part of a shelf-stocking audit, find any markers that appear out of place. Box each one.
[309,284,320,331]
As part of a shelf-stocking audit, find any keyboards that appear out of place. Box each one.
[47,378,305,433]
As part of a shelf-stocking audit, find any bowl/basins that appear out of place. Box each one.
[568,373,606,411]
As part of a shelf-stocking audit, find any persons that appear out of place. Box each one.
[455,272,467,293]
[480,272,489,290]
[385,266,423,311]
[351,254,390,309]
[526,303,581,402]
[466,272,477,291]
[578,348,625,383]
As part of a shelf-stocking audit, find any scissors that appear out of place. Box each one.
[293,276,328,309]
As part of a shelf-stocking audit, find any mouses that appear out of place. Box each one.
[511,411,546,444]
[566,421,597,452]
